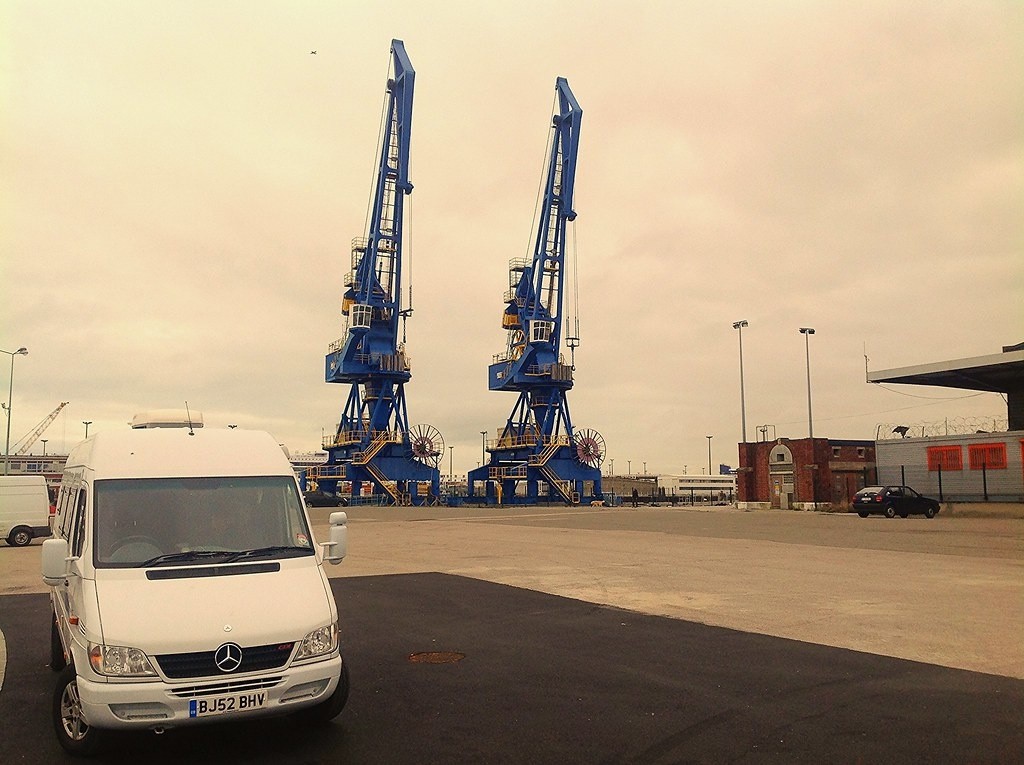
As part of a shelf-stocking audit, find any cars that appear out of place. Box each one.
[851,484,941,519]
[301,490,349,508]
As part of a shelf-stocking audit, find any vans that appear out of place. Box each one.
[40,411,351,757]
[0,475,54,547]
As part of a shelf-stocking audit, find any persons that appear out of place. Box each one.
[632,488,639,507]
[720,491,726,501]
[615,496,623,507]
[47,484,54,500]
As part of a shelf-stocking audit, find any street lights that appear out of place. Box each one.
[479,431,487,466]
[0,347,29,476]
[798,326,816,439]
[643,462,646,474]
[610,458,614,475]
[627,461,631,474]
[448,446,454,481]
[706,435,714,506]
[608,463,611,475]
[732,319,749,443]
[41,439,49,457]
[702,467,705,475]
[684,464,687,475]
[82,421,93,439]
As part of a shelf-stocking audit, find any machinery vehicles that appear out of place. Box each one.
[0,401,70,468]
